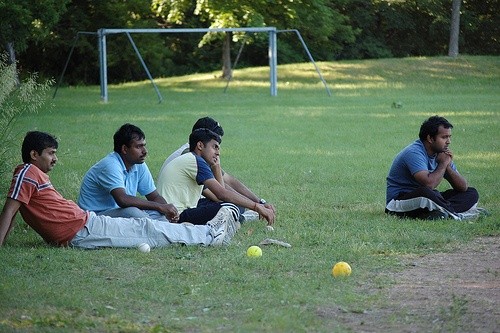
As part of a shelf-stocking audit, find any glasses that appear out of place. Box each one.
[211,122,220,130]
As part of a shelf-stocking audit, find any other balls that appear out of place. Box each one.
[138,242,150,253]
[333,262,352,277]
[247,246,263,259]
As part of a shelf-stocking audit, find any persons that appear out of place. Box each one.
[156,117,278,226]
[0,132,236,258]
[77,123,179,223]
[386,115,490,220]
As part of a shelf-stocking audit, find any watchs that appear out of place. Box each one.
[259,199,266,204]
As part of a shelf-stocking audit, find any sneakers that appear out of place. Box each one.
[472,207,489,217]
[457,213,479,224]
[205,206,229,248]
[242,208,261,223]
